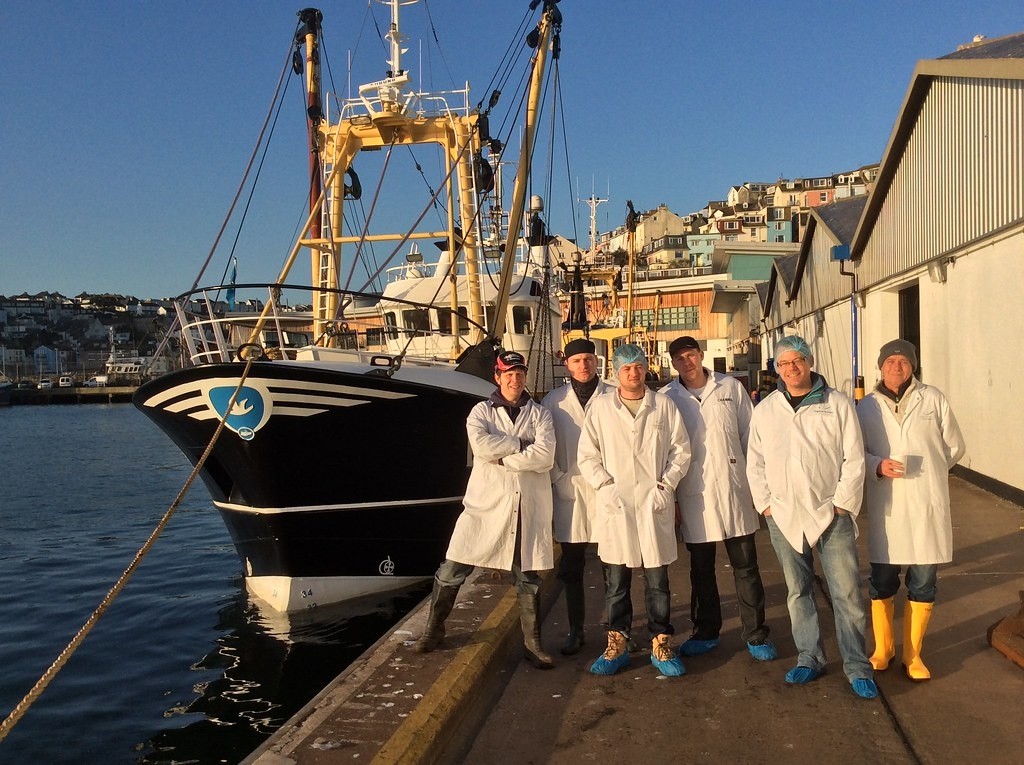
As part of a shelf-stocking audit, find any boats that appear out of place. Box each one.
[131,0,583,615]
[562,170,666,392]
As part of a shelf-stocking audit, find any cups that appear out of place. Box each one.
[890,454,907,475]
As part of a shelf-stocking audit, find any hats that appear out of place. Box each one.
[878,338,917,372]
[497,351,528,371]
[669,336,701,359]
[564,338,595,358]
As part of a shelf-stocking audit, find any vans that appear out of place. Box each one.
[59,377,72,387]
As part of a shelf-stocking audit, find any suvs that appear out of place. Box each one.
[37,379,52,389]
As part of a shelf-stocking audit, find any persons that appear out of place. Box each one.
[577,344,691,676]
[541,339,637,655]
[855,340,966,682]
[746,335,878,699]
[413,351,556,668]
[656,336,777,660]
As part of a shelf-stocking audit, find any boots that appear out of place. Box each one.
[868,595,897,675]
[902,597,934,682]
[412,576,461,654]
[589,631,630,675]
[518,593,556,669]
[651,634,685,677]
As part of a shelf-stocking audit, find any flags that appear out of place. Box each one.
[226,259,237,312]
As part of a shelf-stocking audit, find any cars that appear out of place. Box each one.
[17,380,32,388]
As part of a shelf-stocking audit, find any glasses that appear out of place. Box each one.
[778,357,805,368]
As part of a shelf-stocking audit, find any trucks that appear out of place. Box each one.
[83,376,107,386]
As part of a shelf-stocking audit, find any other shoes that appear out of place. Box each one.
[561,623,587,655]
[747,634,778,661]
[784,664,823,684]
[679,632,720,657]
[851,678,878,699]
